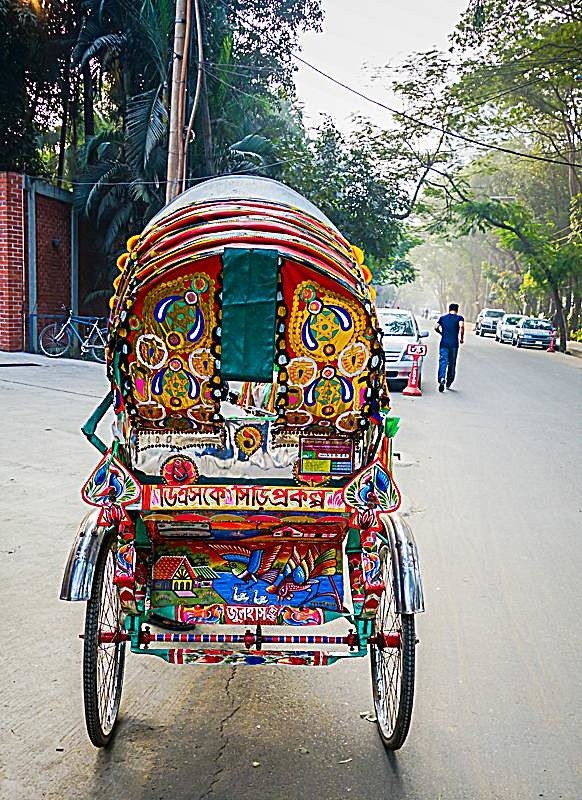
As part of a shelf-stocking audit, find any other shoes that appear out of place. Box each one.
[446,380,452,387]
[439,378,445,392]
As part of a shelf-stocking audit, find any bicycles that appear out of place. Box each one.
[39,305,109,364]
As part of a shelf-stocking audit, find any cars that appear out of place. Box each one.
[495,314,529,345]
[510,318,557,348]
[375,307,430,390]
[430,310,441,319]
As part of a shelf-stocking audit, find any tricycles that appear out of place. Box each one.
[59,173,429,748]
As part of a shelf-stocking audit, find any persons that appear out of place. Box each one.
[434,304,464,392]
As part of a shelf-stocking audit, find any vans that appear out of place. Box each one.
[474,308,506,336]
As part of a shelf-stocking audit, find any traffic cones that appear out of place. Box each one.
[401,352,422,396]
[545,335,557,352]
